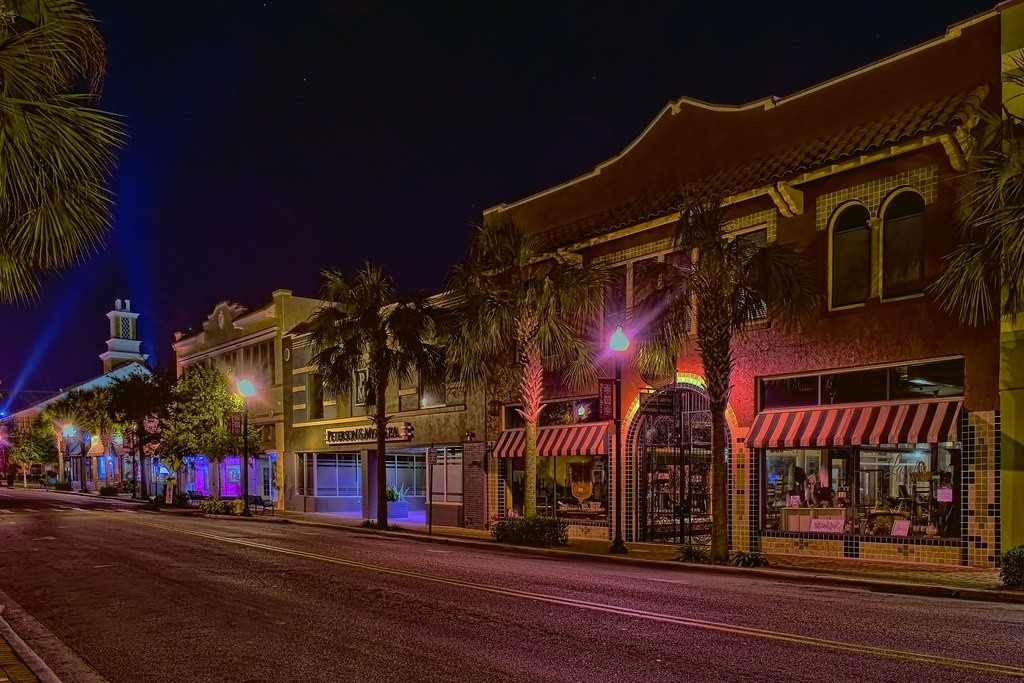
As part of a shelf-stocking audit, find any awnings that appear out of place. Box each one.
[744,400,964,448]
[87,443,104,455]
[491,425,609,456]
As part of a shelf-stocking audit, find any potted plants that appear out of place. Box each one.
[386,482,410,518]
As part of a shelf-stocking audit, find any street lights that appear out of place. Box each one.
[608,325,631,554]
[237,379,255,517]
[63,424,75,491]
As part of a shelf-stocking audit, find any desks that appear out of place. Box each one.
[782,508,846,532]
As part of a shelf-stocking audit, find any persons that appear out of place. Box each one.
[803,472,817,507]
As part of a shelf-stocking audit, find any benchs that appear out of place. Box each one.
[187,490,209,506]
[243,495,275,516]
